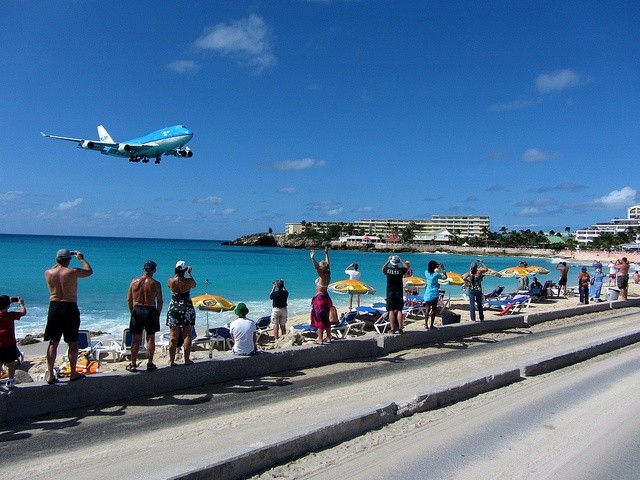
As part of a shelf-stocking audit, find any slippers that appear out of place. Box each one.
[324,339,331,343]
[185,361,193,365]
[313,340,323,345]
[70,373,85,381]
[46,375,55,384]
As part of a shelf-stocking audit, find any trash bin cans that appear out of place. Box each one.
[606,288,620,303]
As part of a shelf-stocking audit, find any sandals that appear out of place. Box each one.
[125,363,136,372]
[147,362,156,371]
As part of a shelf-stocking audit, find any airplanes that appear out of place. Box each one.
[40,124,194,164]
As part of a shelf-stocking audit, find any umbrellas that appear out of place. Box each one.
[402,276,427,290]
[525,265,550,275]
[190,293,236,336]
[327,279,376,312]
[462,267,502,293]
[438,270,465,306]
[497,266,530,295]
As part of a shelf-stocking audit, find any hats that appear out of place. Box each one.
[176,261,187,270]
[234,303,249,316]
[56,249,71,259]
[581,267,587,271]
[390,256,400,265]
[405,261,410,264]
[144,260,157,272]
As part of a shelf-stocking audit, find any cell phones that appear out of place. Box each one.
[476,259,481,262]
[9,297,20,302]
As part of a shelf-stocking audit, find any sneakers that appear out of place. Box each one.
[398,329,403,334]
[5,381,14,388]
[388,330,395,334]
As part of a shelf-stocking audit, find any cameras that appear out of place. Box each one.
[70,251,77,256]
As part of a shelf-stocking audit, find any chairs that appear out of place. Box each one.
[371,301,430,319]
[463,285,505,310]
[291,310,365,339]
[404,294,449,314]
[256,315,273,342]
[66,330,109,361]
[158,331,226,354]
[519,280,552,302]
[486,295,532,316]
[111,328,149,362]
[352,305,388,336]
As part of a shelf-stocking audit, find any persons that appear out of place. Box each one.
[607,260,617,286]
[556,261,569,297]
[310,246,332,345]
[381,255,409,334]
[43,248,94,383]
[517,261,529,289]
[435,263,447,305]
[0,295,26,388]
[229,303,258,356]
[592,258,603,272]
[578,266,590,305]
[530,277,542,297]
[166,261,197,366]
[632,271,640,283]
[462,260,489,322]
[614,257,629,299]
[589,269,606,300]
[329,301,340,325]
[125,259,163,371]
[345,263,361,309]
[403,260,413,295]
[424,260,447,329]
[269,279,289,339]
